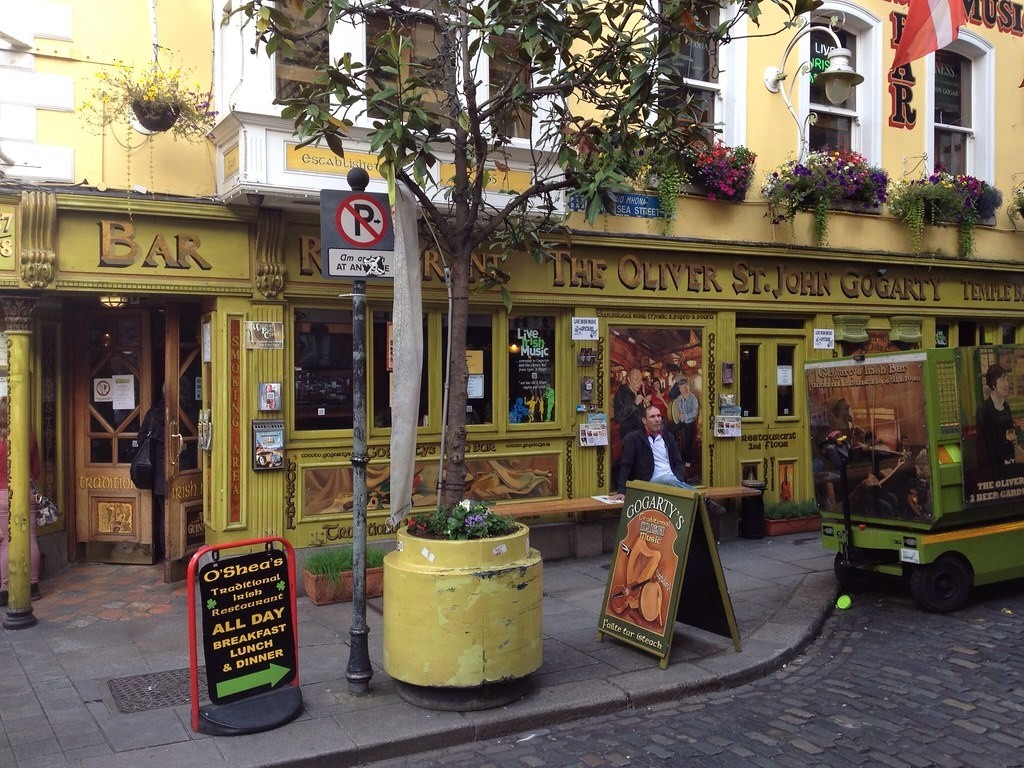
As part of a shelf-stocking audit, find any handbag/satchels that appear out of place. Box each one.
[31,480,59,527]
[130,437,155,490]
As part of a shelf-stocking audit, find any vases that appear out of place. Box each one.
[129,101,181,131]
[382,525,543,711]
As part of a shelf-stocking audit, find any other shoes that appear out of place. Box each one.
[704,495,726,516]
[0,590,8,606]
[30,582,41,600]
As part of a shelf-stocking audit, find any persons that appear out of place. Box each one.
[813,397,919,514]
[607,406,726,518]
[613,368,698,467]
[136,381,166,555]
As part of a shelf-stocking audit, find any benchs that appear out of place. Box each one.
[489,479,765,540]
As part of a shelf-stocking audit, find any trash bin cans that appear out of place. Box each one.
[738,480,767,539]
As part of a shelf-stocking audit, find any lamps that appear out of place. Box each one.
[98,293,129,308]
[763,8,864,165]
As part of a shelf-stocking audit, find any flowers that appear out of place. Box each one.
[72,46,220,225]
[569,124,758,238]
[1005,178,1024,232]
[885,170,1003,283]
[406,498,518,540]
[759,145,891,256]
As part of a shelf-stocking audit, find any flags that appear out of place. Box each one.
[891,0,968,69]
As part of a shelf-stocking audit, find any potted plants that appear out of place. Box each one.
[300,545,387,605]
[764,497,823,537]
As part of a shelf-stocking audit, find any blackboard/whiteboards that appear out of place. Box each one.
[199,550,297,706]
